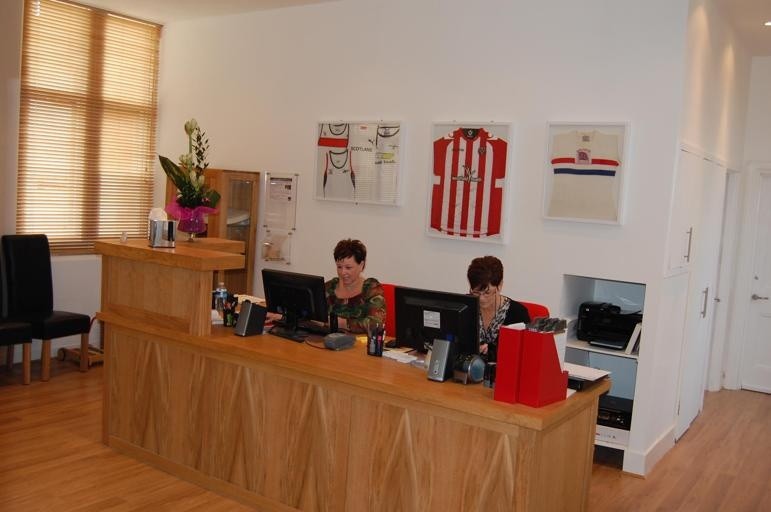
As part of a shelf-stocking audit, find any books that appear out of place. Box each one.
[624,322,642,355]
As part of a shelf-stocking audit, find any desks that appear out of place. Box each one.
[95,237,614,512]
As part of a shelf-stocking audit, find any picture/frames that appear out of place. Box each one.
[540,122,630,226]
[314,120,404,206]
[262,171,299,231]
[425,120,515,246]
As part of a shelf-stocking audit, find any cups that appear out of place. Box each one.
[485,362,496,384]
[224,308,235,326]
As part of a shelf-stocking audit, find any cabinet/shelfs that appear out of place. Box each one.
[555,261,688,479]
[165,166,261,295]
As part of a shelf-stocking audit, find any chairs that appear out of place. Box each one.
[0,234,91,382]
[376,283,406,341]
[0,239,33,385]
[519,301,553,323]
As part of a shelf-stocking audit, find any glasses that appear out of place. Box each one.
[470,288,497,297]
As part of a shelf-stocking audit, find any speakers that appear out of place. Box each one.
[234,301,267,336]
[427,339,455,382]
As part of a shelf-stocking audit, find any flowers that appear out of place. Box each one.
[158,118,221,209]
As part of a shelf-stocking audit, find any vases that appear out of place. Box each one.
[163,197,221,242]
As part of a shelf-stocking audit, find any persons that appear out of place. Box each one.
[464,256,531,373]
[264,238,387,334]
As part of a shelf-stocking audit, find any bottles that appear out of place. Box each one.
[215,282,227,311]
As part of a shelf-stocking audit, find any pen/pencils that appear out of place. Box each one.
[367,322,386,355]
[222,300,237,312]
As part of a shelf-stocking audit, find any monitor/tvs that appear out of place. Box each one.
[395,286,479,370]
[262,268,328,343]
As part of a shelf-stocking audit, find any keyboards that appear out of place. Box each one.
[272,319,330,336]
